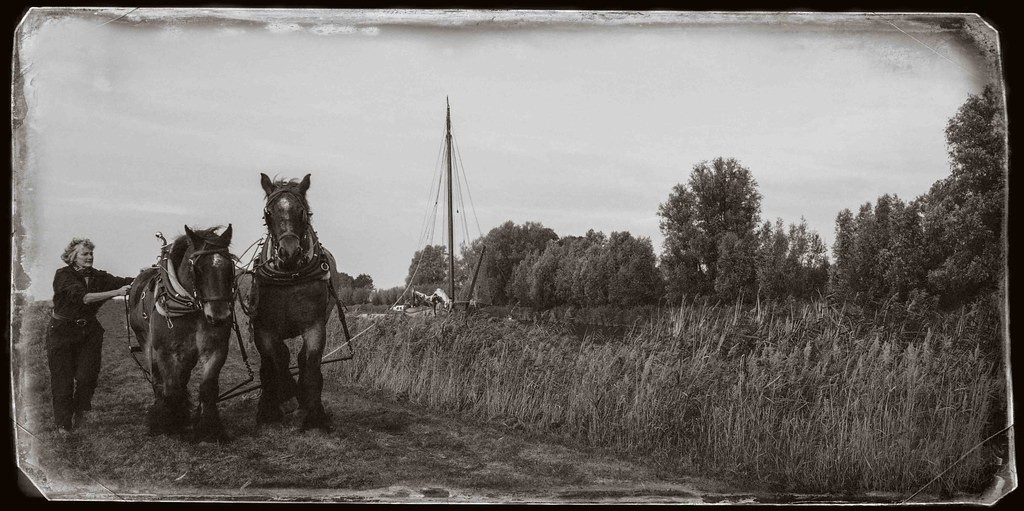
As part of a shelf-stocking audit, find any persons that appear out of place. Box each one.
[432,294,442,317]
[45,239,135,435]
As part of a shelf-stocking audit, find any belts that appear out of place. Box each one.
[52,309,96,328]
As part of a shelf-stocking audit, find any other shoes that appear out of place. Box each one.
[59,425,72,438]
[72,410,84,428]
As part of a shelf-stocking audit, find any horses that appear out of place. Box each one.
[124,221,237,446]
[249,171,339,437]
